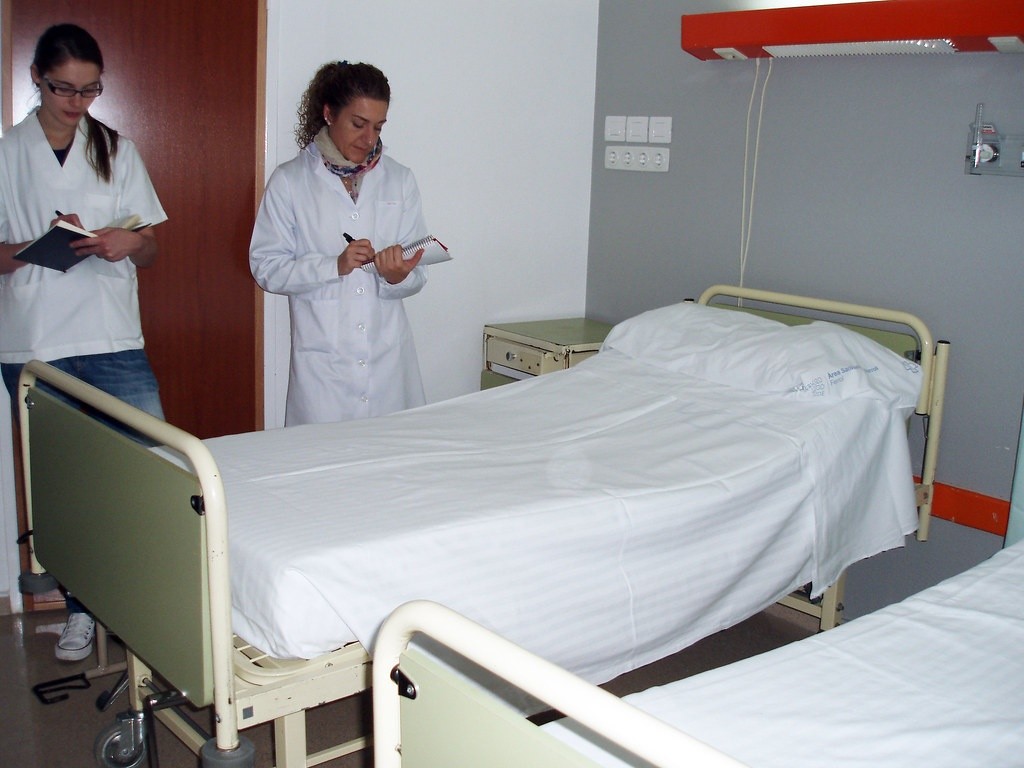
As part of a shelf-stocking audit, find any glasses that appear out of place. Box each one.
[37,68,103,98]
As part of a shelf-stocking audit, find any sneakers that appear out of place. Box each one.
[55,612,96,661]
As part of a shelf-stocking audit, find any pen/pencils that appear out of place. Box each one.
[343,232,356,244]
[55,208,66,218]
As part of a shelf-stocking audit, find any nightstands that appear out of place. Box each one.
[480,318,614,391]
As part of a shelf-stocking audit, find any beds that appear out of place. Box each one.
[18,286,951,768]
[374,409,1024,768]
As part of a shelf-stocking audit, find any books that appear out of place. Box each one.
[13,214,152,272]
[360,235,454,274]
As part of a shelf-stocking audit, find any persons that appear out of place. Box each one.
[248,60,429,428]
[0,22,168,661]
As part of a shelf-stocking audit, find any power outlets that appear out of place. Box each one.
[604,146,671,173]
